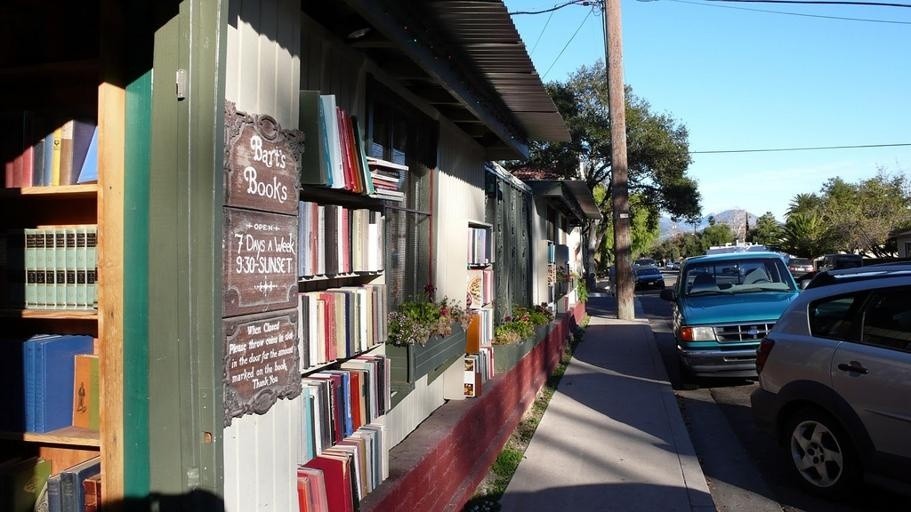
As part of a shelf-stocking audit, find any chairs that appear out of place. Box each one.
[690,270,773,292]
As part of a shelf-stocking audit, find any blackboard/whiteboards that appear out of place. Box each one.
[224,101,306,425]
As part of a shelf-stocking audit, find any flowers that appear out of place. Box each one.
[387,281,474,349]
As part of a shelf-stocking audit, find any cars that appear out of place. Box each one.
[633,256,666,291]
[668,250,864,386]
[749,261,911,497]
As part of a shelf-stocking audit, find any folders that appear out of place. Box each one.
[22,335,94,433]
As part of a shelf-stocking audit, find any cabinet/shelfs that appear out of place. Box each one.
[300,180,390,512]
[0,59,125,512]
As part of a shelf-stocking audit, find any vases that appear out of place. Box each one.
[384,323,467,386]
